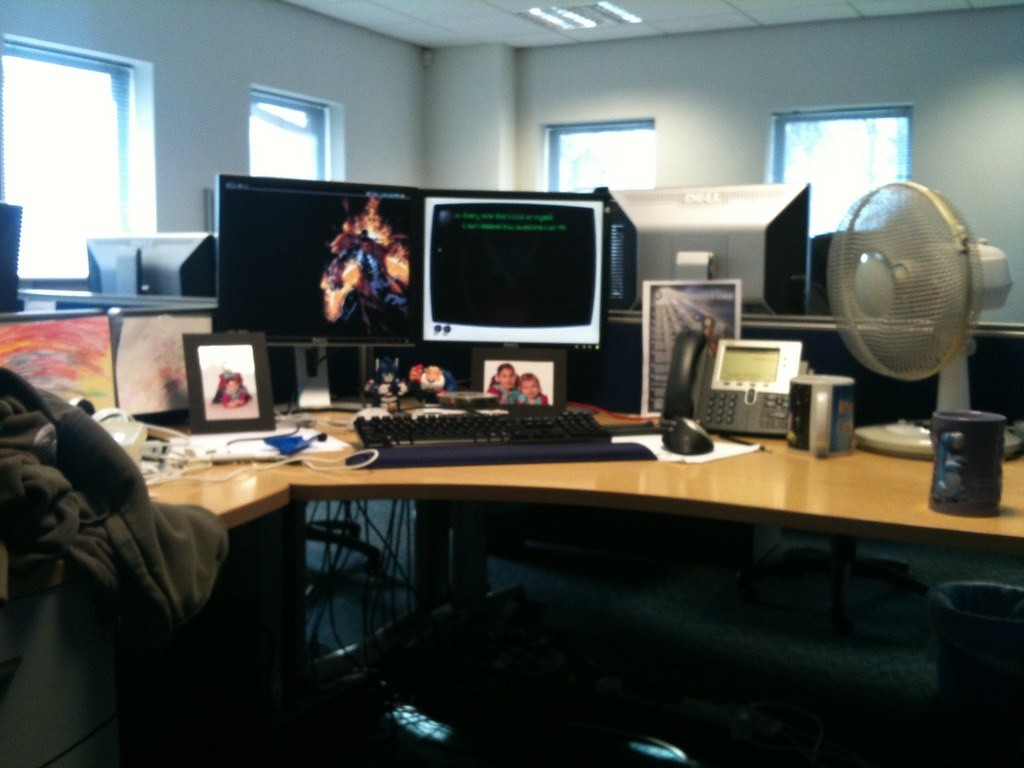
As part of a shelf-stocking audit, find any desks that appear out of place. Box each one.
[106,404,1024,768]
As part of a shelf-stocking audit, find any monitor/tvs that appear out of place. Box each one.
[87,173,810,416]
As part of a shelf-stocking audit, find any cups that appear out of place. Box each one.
[785,375,854,459]
[927,409,1006,518]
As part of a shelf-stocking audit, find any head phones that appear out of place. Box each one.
[69,397,192,467]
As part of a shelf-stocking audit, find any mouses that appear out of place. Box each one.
[663,418,713,455]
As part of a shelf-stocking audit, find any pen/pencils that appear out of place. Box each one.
[718,434,774,453]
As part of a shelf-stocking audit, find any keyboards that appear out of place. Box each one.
[354,411,610,450]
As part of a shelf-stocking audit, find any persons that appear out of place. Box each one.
[213,371,253,409]
[486,364,548,406]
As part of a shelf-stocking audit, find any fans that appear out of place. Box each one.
[823,179,1024,461]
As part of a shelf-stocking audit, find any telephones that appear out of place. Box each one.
[661,329,805,437]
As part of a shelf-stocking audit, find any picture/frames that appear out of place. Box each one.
[181,331,275,435]
[470,346,568,414]
